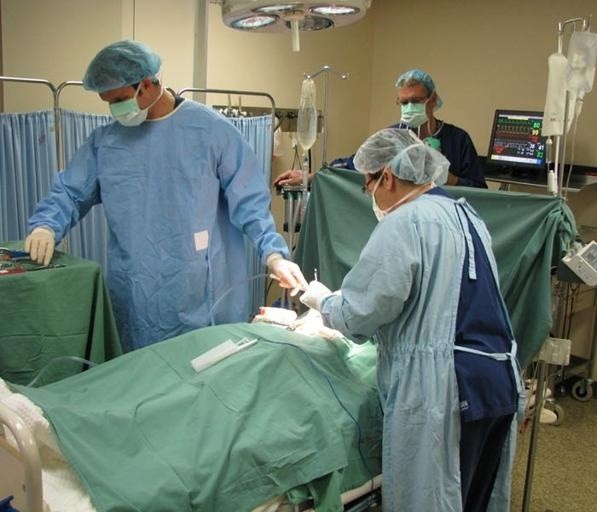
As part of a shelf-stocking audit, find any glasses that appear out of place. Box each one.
[361,171,383,198]
[396,91,427,107]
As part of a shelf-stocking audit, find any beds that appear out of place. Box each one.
[0,381,382,512]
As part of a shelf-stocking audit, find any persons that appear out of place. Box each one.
[24,38,312,357]
[297,127,529,512]
[271,68,494,196]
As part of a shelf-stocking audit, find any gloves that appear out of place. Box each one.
[267,253,307,296]
[299,281,333,312]
[24,227,55,266]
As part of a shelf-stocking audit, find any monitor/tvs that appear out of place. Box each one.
[486,109,550,182]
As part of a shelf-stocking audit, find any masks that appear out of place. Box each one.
[373,193,386,220]
[108,98,148,129]
[400,104,427,129]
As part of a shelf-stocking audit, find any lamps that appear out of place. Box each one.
[208,0,373,53]
